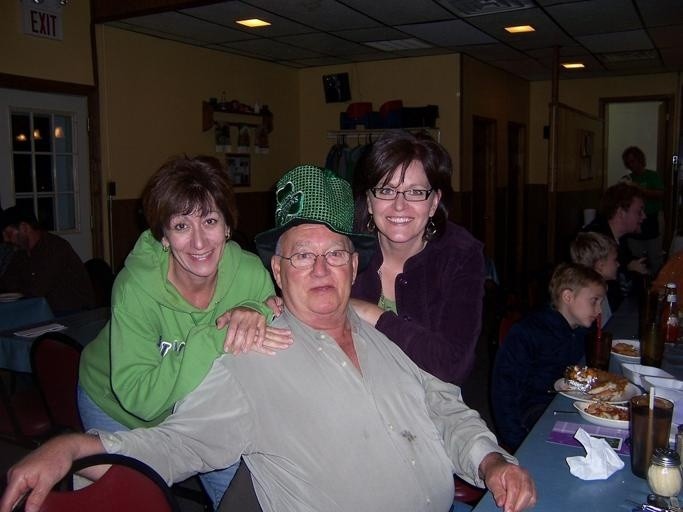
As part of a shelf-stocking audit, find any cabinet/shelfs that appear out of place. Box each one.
[202,99,273,148]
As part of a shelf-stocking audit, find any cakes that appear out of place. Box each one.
[588,401,627,421]
[612,342,638,356]
[565,364,630,398]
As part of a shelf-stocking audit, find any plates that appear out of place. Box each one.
[553,371,642,403]
[572,400,632,430]
[611,337,643,359]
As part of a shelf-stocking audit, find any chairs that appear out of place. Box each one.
[0,332,184,512]
[84,257,116,308]
[485,263,560,456]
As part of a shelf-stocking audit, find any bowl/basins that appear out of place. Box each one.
[620,361,683,424]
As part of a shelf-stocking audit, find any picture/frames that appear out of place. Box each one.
[224,153,251,187]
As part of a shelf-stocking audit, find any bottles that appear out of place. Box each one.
[641,282,678,364]
[585,313,609,369]
[646,447,681,497]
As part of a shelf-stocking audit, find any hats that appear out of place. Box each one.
[254,165,376,250]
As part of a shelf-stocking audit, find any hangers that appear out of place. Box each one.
[327,133,375,159]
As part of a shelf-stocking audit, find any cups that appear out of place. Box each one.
[629,394,674,479]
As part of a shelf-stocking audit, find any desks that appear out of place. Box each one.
[1,296,109,393]
[470,286,683,511]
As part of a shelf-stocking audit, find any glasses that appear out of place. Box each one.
[371,188,434,201]
[276,250,352,270]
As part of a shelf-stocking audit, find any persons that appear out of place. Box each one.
[0,221,540,512]
[490,263,609,455]
[74,156,293,436]
[265,134,493,437]
[568,145,666,328]
[0,204,96,319]
